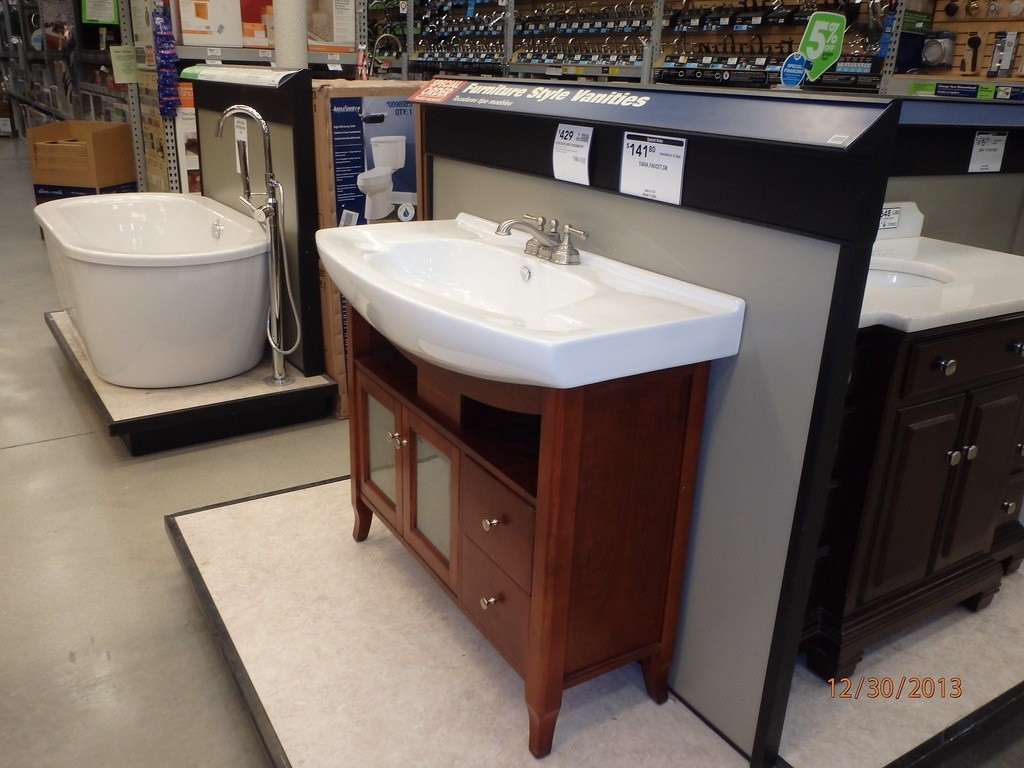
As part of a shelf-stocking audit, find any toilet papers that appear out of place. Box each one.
[271,0,308,69]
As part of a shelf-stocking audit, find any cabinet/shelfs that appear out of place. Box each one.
[324,294,705,759]
[796,312,1024,683]
[0,0,144,194]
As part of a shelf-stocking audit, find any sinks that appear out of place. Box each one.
[313,219,641,389]
[865,257,959,290]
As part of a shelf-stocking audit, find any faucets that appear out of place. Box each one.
[215,103,280,223]
[365,0,889,78]
[496,217,560,260]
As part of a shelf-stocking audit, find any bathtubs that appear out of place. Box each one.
[32,192,273,390]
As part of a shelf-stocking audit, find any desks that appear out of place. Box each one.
[26,117,138,240]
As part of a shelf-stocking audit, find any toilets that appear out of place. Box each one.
[356,136,408,220]
